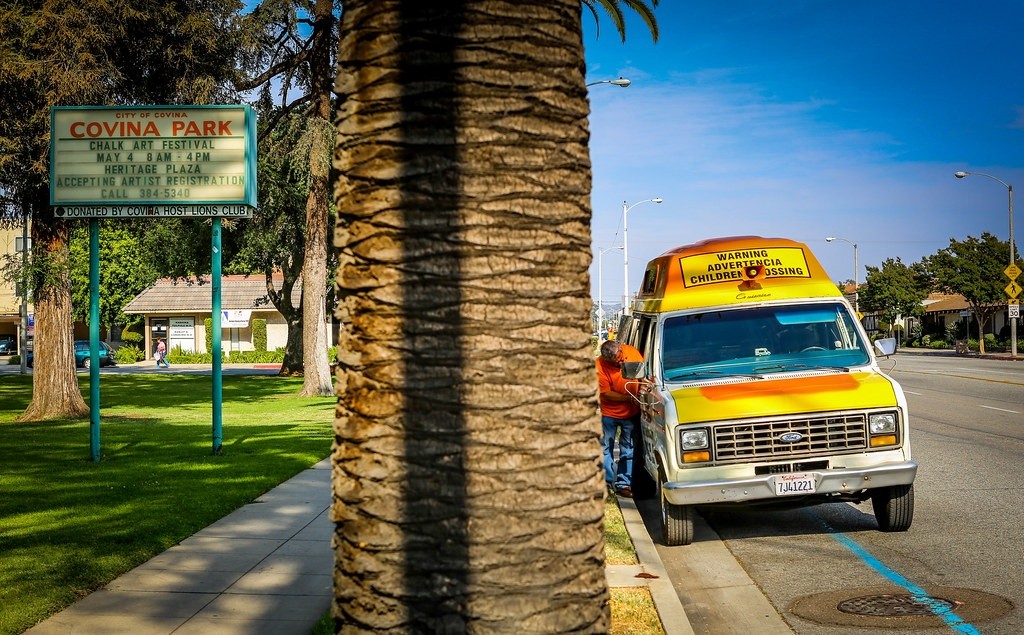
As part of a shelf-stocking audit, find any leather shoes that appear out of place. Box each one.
[616,486,634,498]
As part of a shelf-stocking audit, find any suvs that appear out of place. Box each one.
[613,236,919,545]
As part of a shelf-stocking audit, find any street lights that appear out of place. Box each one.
[826,238,859,348]
[955,172,1018,358]
[598,247,624,342]
[623,197,663,315]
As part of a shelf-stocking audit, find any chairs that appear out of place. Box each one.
[780,328,815,354]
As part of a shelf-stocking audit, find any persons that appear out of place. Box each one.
[595,340,644,496]
[156,336,170,369]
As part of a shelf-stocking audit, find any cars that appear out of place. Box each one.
[27,339,33,353]
[27,341,116,369]
[0,340,16,355]
